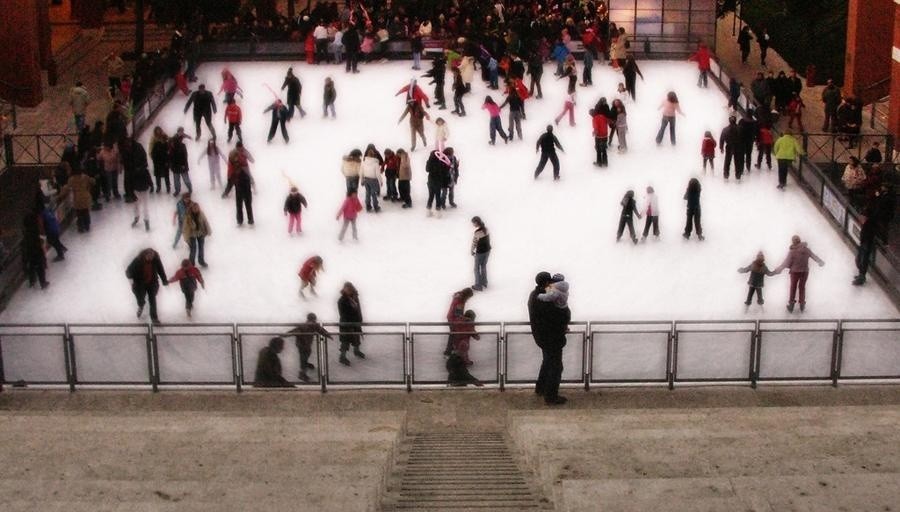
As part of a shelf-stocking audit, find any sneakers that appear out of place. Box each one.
[544,395,567,405]
[353,348,365,358]
[799,301,806,311]
[786,302,795,312]
[851,275,866,284]
[338,352,351,366]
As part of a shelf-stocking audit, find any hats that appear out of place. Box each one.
[756,251,764,262]
[183,193,190,198]
[553,273,564,281]
[791,234,802,244]
